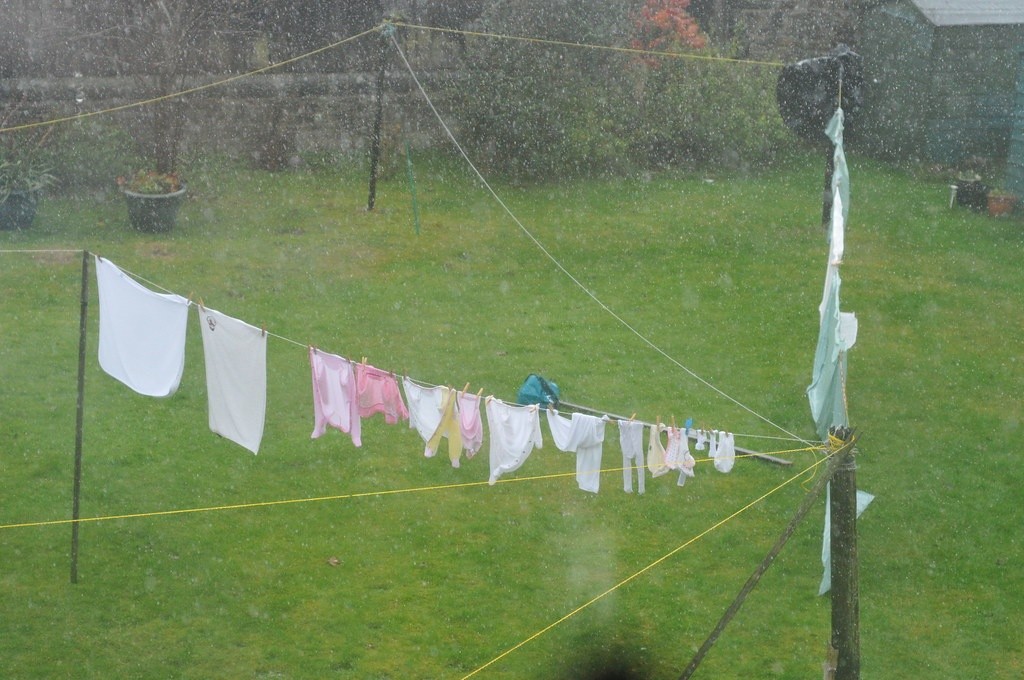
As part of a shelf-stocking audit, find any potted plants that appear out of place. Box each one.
[117,169,187,233]
[985,187,1021,219]
[0,127,62,232]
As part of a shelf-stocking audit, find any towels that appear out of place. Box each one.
[93,255,188,401]
[196,303,269,457]
[545,411,608,491]
